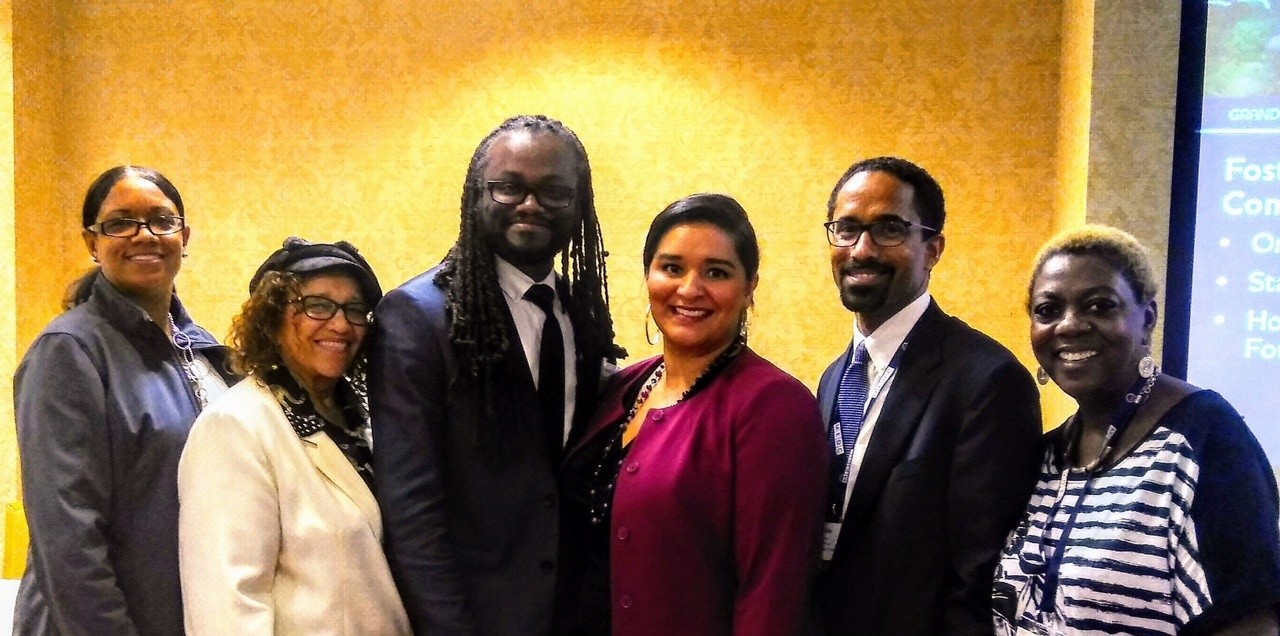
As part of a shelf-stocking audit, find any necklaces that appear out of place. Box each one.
[588,357,718,523]
[1062,363,1161,476]
[163,309,212,407]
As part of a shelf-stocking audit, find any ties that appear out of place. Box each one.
[824,340,869,523]
[521,285,565,463]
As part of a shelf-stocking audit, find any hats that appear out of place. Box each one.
[247,244,382,306]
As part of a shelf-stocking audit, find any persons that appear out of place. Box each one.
[176,235,417,636]
[366,115,618,636]
[815,157,1042,636]
[562,191,830,636]
[989,222,1279,636]
[11,165,247,636]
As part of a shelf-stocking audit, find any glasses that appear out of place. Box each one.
[824,219,937,247]
[480,179,584,210]
[86,216,184,238]
[280,296,376,327]
